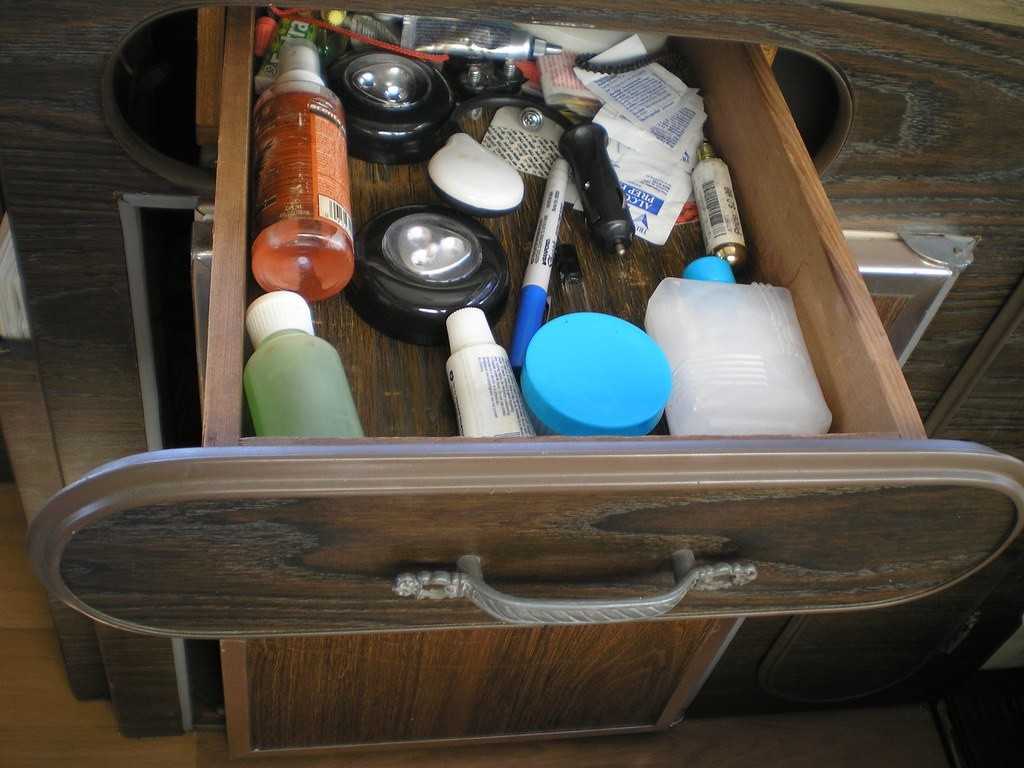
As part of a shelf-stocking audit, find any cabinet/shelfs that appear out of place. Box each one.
[0,0,1024,760]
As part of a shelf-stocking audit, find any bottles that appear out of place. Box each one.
[245,290,365,440]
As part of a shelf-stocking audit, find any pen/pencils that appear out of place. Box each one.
[509,157,570,367]
